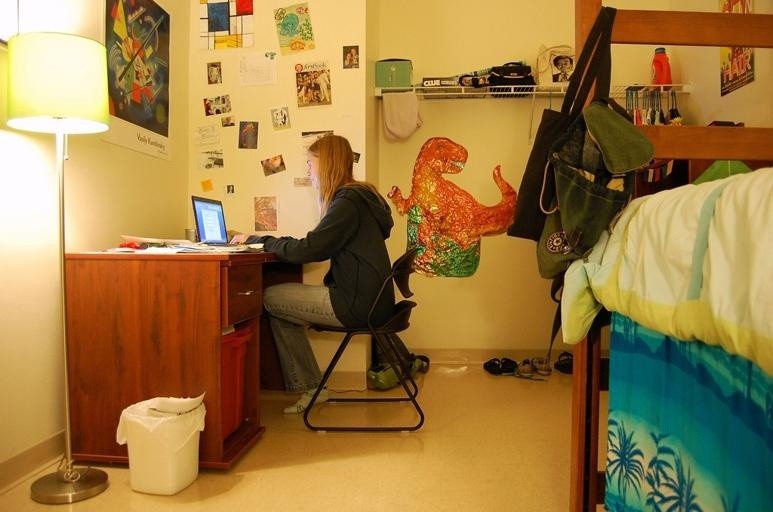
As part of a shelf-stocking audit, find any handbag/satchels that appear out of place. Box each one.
[490,6,655,279]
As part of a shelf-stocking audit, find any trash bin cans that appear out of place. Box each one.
[115,396,206,497]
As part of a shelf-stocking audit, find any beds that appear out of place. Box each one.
[571,0,773,512]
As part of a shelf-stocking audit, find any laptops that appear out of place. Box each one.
[191,195,241,247]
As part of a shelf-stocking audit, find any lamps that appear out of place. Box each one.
[1,31,110,505]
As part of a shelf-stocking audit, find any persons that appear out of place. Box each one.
[274,109,288,126]
[552,55,573,82]
[204,99,213,115]
[296,70,331,104]
[264,159,272,176]
[225,95,233,112]
[229,135,395,414]
[243,125,256,147]
[223,117,235,126]
[215,96,221,105]
[222,96,225,104]
[345,48,358,67]
[214,107,222,114]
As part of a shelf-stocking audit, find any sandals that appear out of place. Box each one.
[554,349,574,375]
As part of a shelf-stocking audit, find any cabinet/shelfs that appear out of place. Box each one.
[64,252,303,471]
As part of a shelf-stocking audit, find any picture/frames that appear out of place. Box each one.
[0,0,20,45]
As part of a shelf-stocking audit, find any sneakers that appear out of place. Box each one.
[518,353,549,383]
[532,354,553,377]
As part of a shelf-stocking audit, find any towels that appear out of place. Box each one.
[382,90,422,144]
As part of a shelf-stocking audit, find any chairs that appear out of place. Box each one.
[303,245,425,432]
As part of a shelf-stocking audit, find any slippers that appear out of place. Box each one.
[484,357,502,375]
[501,357,517,375]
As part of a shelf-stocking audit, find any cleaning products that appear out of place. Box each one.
[650,48,671,91]
